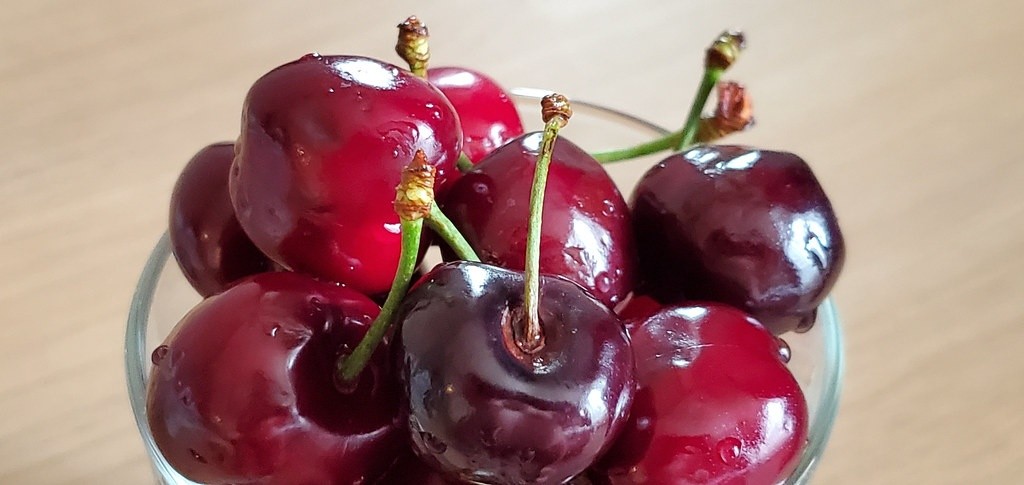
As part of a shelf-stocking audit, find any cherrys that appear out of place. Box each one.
[143,15,846,485]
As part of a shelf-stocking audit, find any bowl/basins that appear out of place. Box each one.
[121,90,841,484]
